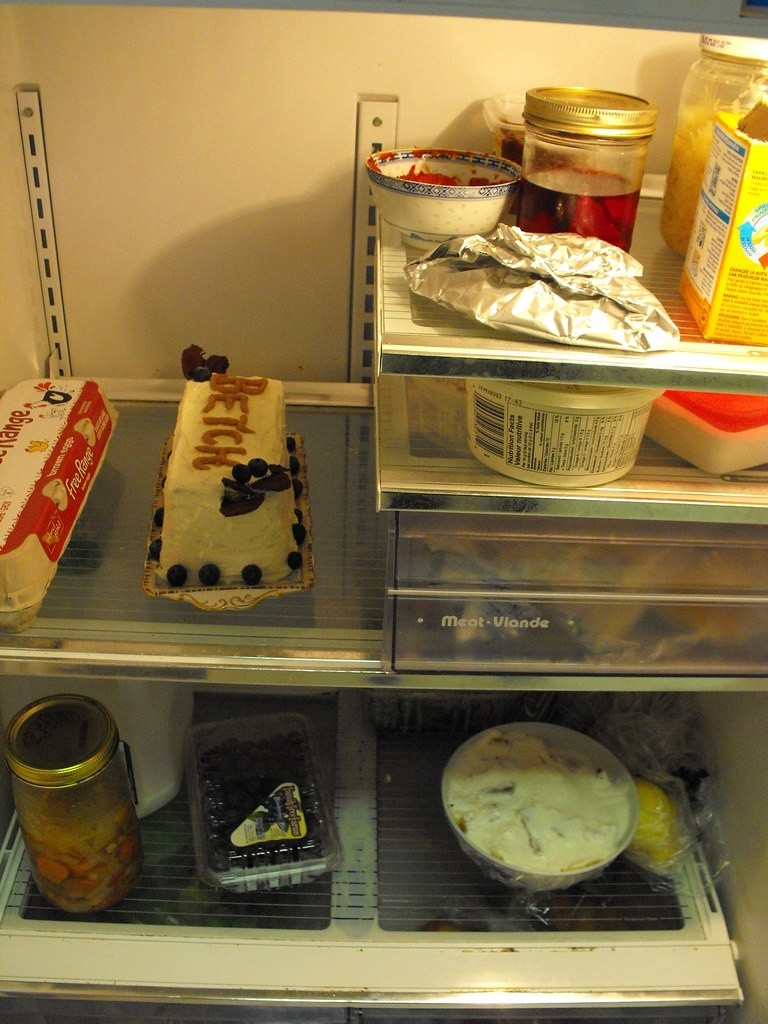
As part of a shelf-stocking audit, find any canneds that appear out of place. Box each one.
[660,33,767,256]
[3,693,145,916]
[516,88,659,252]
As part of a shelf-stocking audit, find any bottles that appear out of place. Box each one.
[481,93,525,167]
[659,33,768,264]
[516,84,656,253]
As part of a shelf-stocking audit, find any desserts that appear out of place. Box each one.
[0,341,305,606]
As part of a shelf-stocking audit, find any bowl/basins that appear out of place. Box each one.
[462,373,667,492]
[184,705,346,894]
[437,716,644,893]
[365,146,524,249]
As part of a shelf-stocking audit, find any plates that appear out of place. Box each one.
[139,427,317,610]
